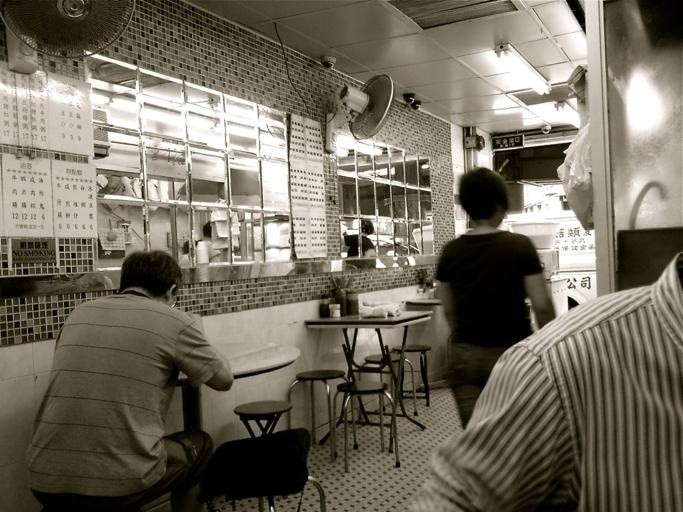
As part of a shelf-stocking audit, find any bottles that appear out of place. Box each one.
[317,294,342,318]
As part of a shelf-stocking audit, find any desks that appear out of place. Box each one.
[165,340,300,456]
[297,299,445,454]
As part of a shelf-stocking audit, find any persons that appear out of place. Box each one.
[400,251,683,512]
[25,248,233,512]
[179,219,235,267]
[436,167,557,429]
[344,218,376,258]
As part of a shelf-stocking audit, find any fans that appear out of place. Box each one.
[0,0,137,74]
[324,69,395,155]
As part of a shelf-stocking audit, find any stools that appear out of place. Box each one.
[232,343,433,512]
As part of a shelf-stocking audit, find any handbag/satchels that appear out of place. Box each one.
[199,427,311,501]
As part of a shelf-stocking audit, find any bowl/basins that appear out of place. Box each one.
[511,222,561,250]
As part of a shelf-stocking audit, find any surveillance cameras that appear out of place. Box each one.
[411,101,421,110]
[403,93,415,104]
[321,56,336,68]
